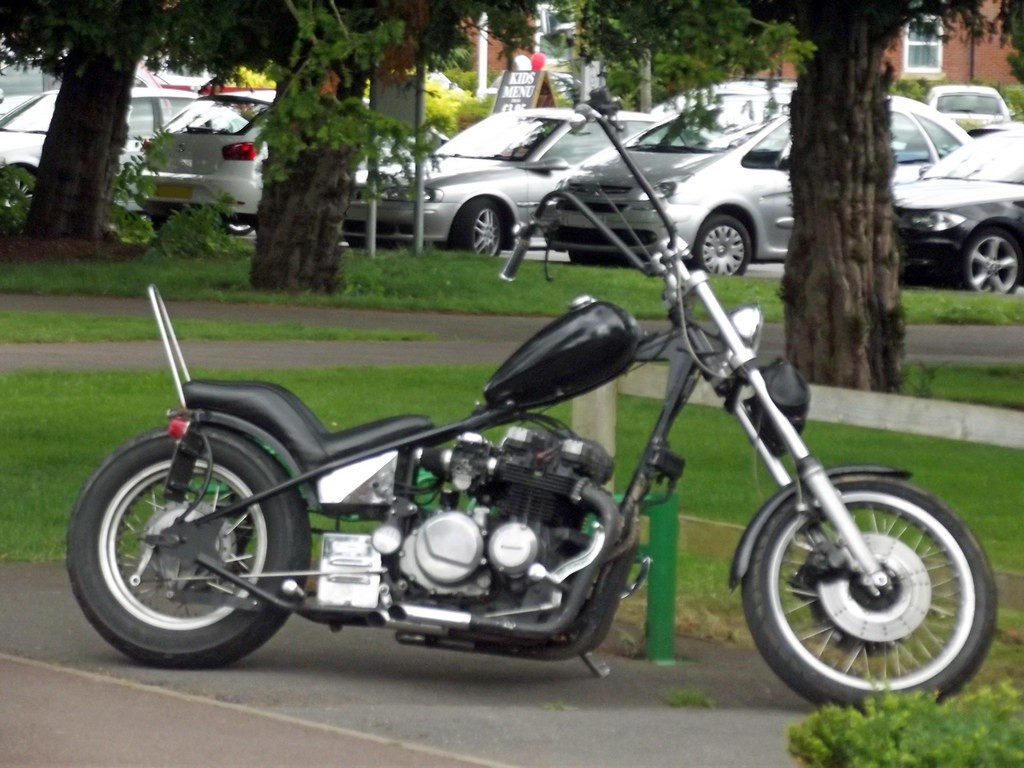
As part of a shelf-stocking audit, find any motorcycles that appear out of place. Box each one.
[66,89,999,718]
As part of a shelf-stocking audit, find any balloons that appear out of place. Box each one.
[512,55,531,70]
[532,53,545,69]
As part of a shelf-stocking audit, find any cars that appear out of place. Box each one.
[128,90,277,237]
[545,93,975,280]
[1,86,198,215]
[891,124,1024,296]
[931,82,1014,138]
[335,107,666,262]
